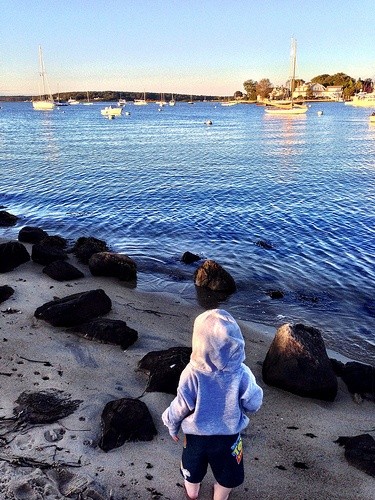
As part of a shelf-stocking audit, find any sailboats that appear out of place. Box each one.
[343,64,375,107]
[81,86,94,105]
[30,43,56,111]
[155,87,177,107]
[262,34,310,115]
[117,93,127,105]
[202,95,208,103]
[132,86,148,106]
[187,90,195,104]
[52,83,69,106]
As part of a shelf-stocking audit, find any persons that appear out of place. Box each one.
[161,309,263,500]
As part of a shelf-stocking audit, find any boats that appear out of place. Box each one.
[99,105,124,116]
[68,97,80,106]
[220,100,236,107]
